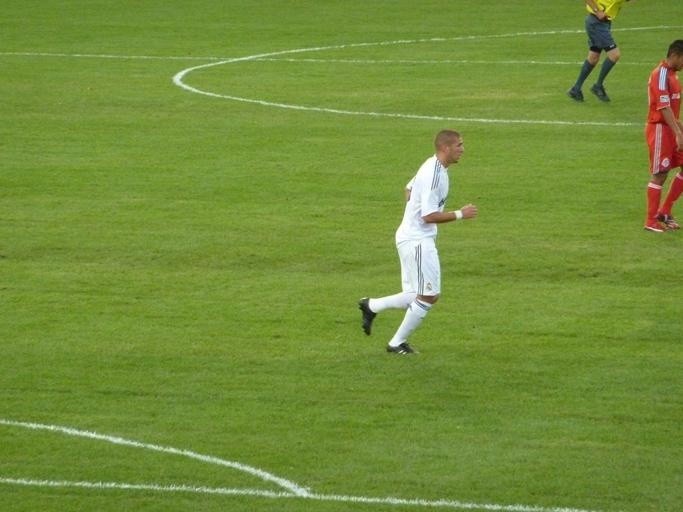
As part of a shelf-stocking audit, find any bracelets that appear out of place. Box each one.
[453,209,464,222]
[592,8,599,13]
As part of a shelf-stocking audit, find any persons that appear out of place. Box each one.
[566,0,630,103]
[357,129,478,356]
[643,39,683,234]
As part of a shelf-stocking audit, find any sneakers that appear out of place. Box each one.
[592,84,609,101]
[358,296,378,335]
[644,208,680,231]
[385,342,415,355]
[568,86,584,102]
